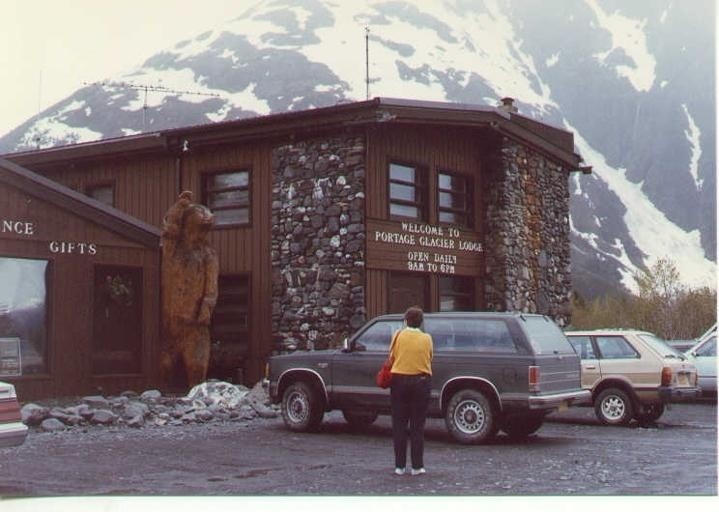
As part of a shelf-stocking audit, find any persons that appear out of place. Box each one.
[389,303,435,476]
[159,188,221,389]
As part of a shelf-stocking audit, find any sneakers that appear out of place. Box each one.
[394,468,426,476]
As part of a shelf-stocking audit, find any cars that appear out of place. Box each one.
[262,312,593,444]
[0,380,29,448]
[563,319,717,427]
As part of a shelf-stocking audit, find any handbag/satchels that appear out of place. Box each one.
[376,362,392,389]
